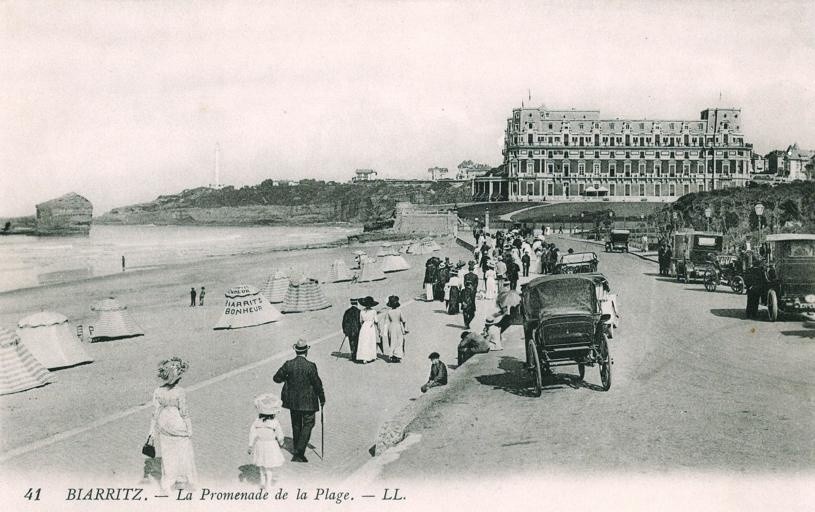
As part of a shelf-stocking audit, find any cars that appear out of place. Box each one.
[753,233,815,322]
[669,232,723,283]
[605,229,630,253]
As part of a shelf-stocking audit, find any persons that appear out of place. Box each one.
[744,259,763,318]
[641,233,648,253]
[149,358,198,489]
[273,339,325,463]
[199,286,206,306]
[247,393,285,488]
[190,287,196,306]
[658,244,672,275]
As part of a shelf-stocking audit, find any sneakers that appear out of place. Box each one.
[292,445,310,463]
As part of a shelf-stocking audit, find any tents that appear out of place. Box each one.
[17,306,94,370]
[328,258,353,284]
[0,323,54,395]
[90,297,144,340]
[213,279,285,330]
[280,272,331,313]
[262,271,289,304]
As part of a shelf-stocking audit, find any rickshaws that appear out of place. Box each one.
[522,250,613,392]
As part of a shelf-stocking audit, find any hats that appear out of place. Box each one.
[386,295,401,308]
[291,338,312,352]
[425,254,504,286]
[252,391,284,415]
[349,297,358,302]
[155,355,189,387]
[483,315,496,324]
[427,351,441,359]
[357,295,380,307]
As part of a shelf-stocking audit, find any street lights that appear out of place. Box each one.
[552,211,556,234]
[754,201,766,246]
[567,213,574,236]
[672,211,678,235]
[580,213,585,237]
[609,208,613,230]
[641,210,645,251]
[705,206,711,233]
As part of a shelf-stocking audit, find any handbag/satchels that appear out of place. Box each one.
[142,434,157,459]
